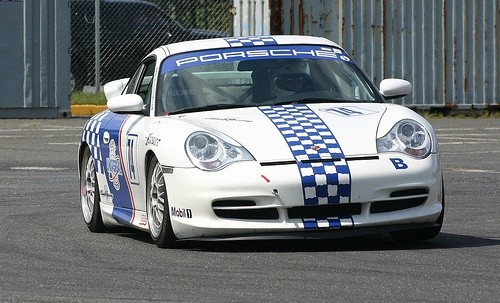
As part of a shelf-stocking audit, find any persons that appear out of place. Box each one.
[270,58,313,102]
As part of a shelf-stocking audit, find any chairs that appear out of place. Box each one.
[165,76,207,112]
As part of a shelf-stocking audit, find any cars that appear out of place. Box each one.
[77,35,445,248]
[71,1,228,83]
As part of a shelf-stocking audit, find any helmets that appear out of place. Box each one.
[269,57,312,98]
